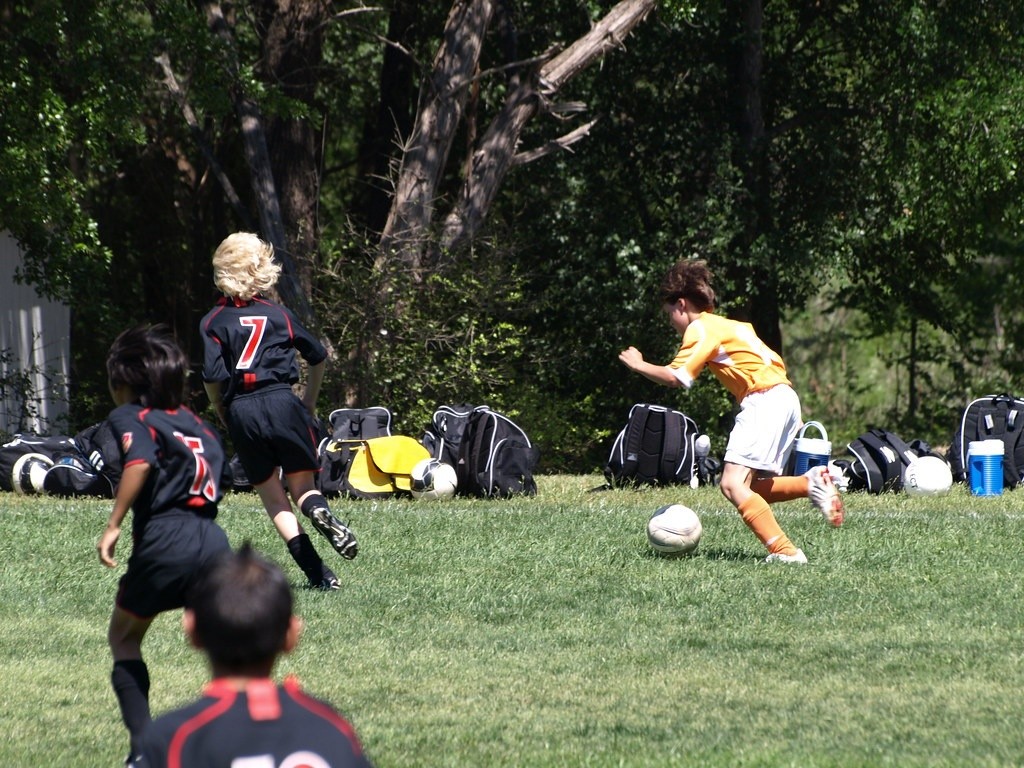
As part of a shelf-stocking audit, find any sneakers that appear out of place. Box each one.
[766,548,808,566]
[804,465,843,528]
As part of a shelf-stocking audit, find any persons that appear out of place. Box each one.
[96,324,229,767]
[619,263,842,564]
[201,232,358,590]
[129,543,370,768]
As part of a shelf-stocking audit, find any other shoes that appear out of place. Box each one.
[310,505,359,560]
[304,571,341,591]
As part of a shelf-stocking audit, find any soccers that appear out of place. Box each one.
[646,505,701,554]
[411,459,458,501]
[903,458,951,498]
[12,453,55,496]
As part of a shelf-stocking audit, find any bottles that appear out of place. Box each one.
[695,435,711,456]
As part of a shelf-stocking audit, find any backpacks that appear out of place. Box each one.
[946,392,1024,491]
[604,404,720,489]
[846,428,920,497]
[315,407,430,501]
[422,405,486,499]
[0,432,115,499]
[455,410,540,502]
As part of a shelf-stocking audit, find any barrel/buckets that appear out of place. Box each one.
[966,440,1005,496]
[794,421,831,476]
